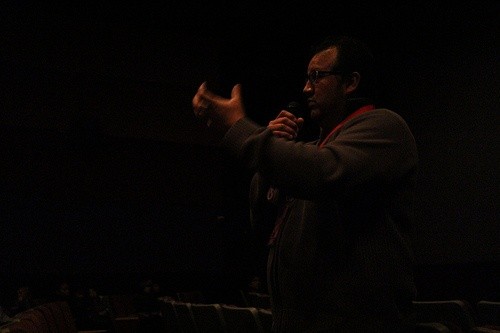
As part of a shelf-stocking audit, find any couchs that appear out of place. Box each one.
[0,294,137,333]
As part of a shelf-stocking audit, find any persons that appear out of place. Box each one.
[192,44,419,333]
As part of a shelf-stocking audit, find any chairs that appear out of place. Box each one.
[158,297,500,333]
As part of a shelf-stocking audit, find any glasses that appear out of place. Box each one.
[306,70,342,82]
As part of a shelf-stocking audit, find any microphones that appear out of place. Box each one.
[287,101,300,118]
